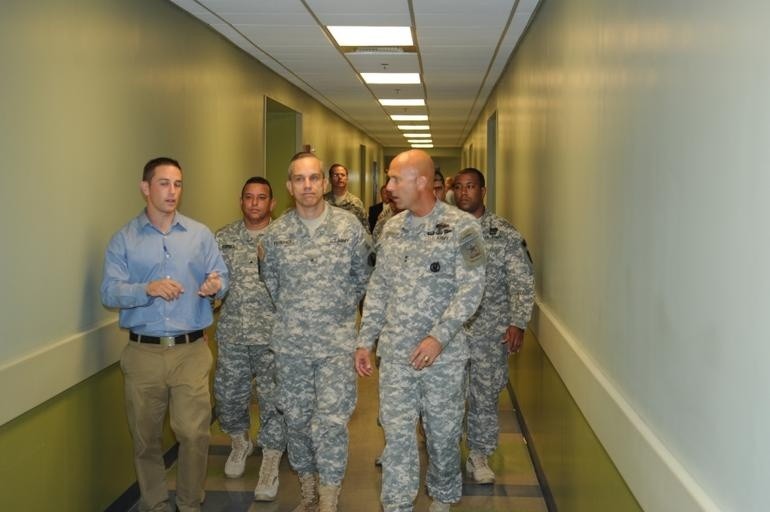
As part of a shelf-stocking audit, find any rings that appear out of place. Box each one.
[425,356,430,362]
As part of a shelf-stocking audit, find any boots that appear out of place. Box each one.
[318,485,341,512]
[465,449,496,485]
[292,472,319,512]
[253,449,283,501]
[427,500,451,512]
[224,431,255,478]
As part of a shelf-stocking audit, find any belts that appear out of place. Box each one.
[130,330,203,347]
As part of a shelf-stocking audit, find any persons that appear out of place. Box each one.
[260,152,371,512]
[450,168,536,485]
[354,149,484,503]
[210,176,282,498]
[100,158,229,511]
[434,166,455,205]
[366,185,398,257]
[325,164,368,233]
